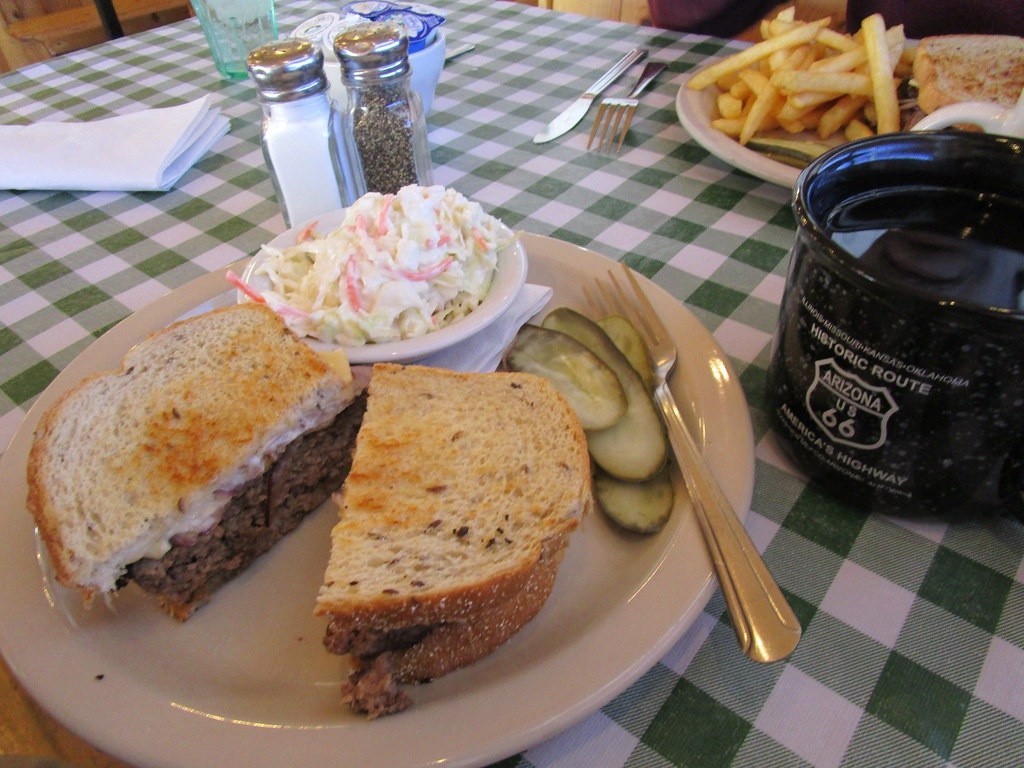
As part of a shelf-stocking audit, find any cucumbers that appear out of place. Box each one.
[503,305,677,538]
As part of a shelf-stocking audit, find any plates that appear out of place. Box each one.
[238,194,527,365]
[677,36,918,187]
[0,228,759,767]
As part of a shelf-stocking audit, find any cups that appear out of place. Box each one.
[188,0,279,79]
[908,82,1023,136]
[768,128,1023,517]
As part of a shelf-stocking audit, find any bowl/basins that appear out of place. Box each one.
[317,26,447,120]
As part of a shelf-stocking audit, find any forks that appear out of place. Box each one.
[582,62,668,153]
[578,260,800,664]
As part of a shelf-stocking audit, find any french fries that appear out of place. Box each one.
[688,5,912,142]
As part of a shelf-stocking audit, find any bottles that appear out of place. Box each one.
[247,38,366,229]
[337,22,436,192]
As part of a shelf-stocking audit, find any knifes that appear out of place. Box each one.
[531,47,648,145]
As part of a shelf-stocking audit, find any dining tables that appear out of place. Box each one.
[2,0,1024,767]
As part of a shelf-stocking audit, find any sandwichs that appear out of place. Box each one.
[912,34,1024,136]
[23,297,589,722]
